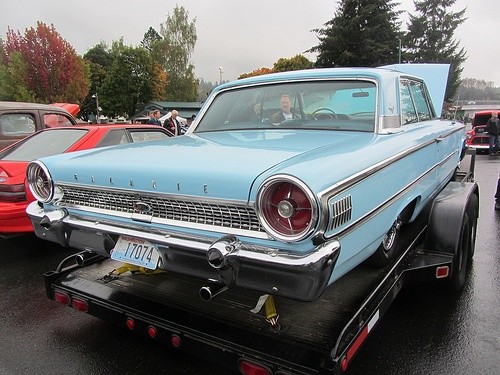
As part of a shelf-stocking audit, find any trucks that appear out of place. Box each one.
[465,109,500,154]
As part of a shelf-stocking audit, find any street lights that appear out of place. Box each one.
[219,66,224,85]
[395,36,402,63]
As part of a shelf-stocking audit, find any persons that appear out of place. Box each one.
[271,94,301,123]
[149,110,162,126]
[486,111,500,157]
[163,110,181,136]
[146,111,154,125]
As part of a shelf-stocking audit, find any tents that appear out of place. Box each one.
[159,112,187,128]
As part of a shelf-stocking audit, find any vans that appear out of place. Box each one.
[0,100,80,151]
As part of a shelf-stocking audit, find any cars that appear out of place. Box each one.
[23,62,469,304]
[0,122,177,235]
